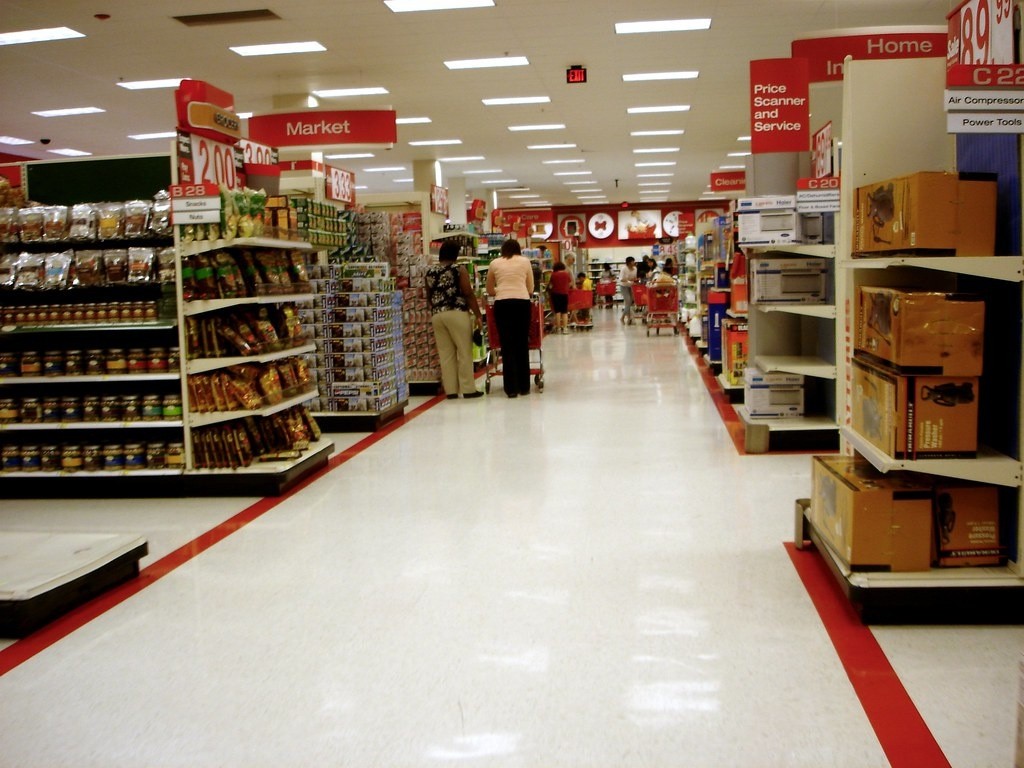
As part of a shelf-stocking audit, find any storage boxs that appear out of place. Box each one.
[255,195,441,413]
[698,172,1009,572]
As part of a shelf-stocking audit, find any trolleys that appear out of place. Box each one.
[628,279,680,337]
[545,290,594,332]
[595,277,618,310]
[481,291,545,395]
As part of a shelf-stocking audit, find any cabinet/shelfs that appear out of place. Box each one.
[313,164,443,396]
[492,209,555,339]
[684,231,696,331]
[588,262,625,303]
[0,153,188,495]
[689,234,701,341]
[695,224,709,356]
[737,82,841,454]
[716,202,747,403]
[703,215,730,376]
[466,199,506,365]
[175,80,334,496]
[794,57,1024,626]
[677,233,688,325]
[239,134,409,431]
[428,184,493,379]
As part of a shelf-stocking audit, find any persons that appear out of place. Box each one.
[485,239,536,397]
[426,241,484,399]
[600,262,614,310]
[579,272,592,290]
[543,262,572,334]
[635,255,675,284]
[618,257,639,325]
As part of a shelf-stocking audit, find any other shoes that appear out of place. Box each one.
[447,394,458,399]
[463,390,484,398]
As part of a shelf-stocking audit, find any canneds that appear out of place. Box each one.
[0,395,183,424]
[0,347,181,378]
[0,301,158,323]
[0,443,186,472]
